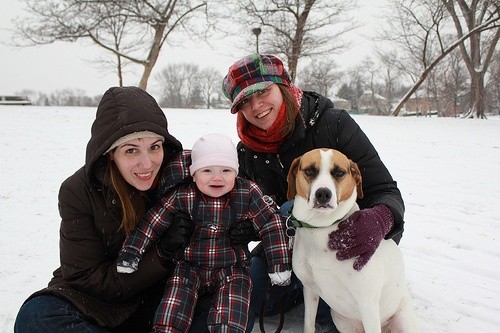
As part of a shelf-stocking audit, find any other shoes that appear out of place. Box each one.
[316,298,332,324]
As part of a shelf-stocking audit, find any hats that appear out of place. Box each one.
[189,133,239,176]
[102,131,165,155]
[222,54,290,114]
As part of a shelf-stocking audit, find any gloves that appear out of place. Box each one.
[327,205,394,270]
[227,219,260,246]
[116,266,135,274]
[156,213,194,259]
[267,271,292,286]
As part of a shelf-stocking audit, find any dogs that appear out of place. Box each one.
[285,145,418,333]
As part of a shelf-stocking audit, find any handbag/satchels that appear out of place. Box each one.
[251,269,304,333]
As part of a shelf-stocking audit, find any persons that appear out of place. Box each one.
[13,86,183,333]
[116,133,293,333]
[220,54,406,333]
